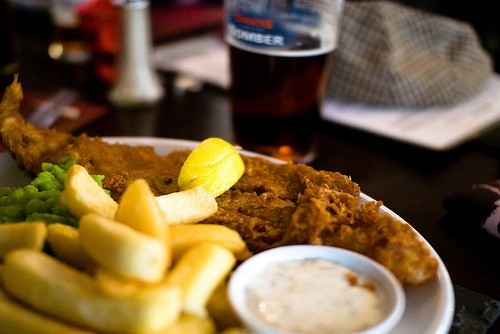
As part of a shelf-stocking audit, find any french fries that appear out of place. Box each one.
[0,164,253,334]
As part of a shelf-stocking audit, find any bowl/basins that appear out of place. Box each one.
[226,244,405,334]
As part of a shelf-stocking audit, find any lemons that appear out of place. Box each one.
[178,137,245,197]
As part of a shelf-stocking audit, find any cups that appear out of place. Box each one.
[222,0,344,163]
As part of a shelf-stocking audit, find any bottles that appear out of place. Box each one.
[107,1,165,105]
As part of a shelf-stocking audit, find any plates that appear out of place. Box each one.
[17,90,106,134]
[0,134,456,334]
